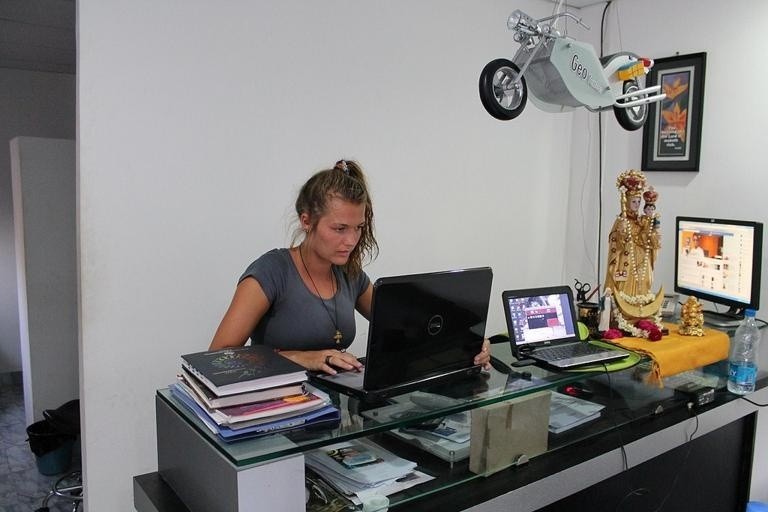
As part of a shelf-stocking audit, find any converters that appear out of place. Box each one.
[673,382,700,401]
[690,387,714,404]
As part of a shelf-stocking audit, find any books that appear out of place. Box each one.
[474,378,606,435]
[180,344,309,409]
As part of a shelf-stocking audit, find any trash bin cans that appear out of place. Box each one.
[26,420,77,476]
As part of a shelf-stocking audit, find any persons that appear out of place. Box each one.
[636,186,661,249]
[683,237,691,256]
[604,168,657,296]
[207,159,492,375]
[688,232,704,257]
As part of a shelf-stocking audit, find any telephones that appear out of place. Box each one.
[661,294,679,317]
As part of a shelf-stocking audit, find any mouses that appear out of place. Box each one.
[557,383,594,399]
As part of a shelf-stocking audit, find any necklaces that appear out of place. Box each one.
[626,214,654,282]
[299,243,347,353]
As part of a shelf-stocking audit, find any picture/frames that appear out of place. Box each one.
[642,55,707,172]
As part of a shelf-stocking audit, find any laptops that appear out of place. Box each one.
[307,267,492,402]
[503,286,630,372]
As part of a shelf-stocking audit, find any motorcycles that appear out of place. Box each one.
[479,2,667,131]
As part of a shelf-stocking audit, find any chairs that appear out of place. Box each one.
[26,396,83,512]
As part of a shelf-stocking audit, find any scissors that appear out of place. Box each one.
[574,279,591,304]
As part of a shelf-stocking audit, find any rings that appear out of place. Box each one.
[325,355,335,366]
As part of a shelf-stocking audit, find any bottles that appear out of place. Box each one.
[578,301,600,340]
[725,309,759,396]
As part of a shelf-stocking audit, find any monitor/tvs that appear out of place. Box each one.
[674,216,763,327]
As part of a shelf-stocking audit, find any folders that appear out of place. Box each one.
[169,383,341,443]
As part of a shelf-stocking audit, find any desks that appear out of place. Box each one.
[133,310,767,512]
